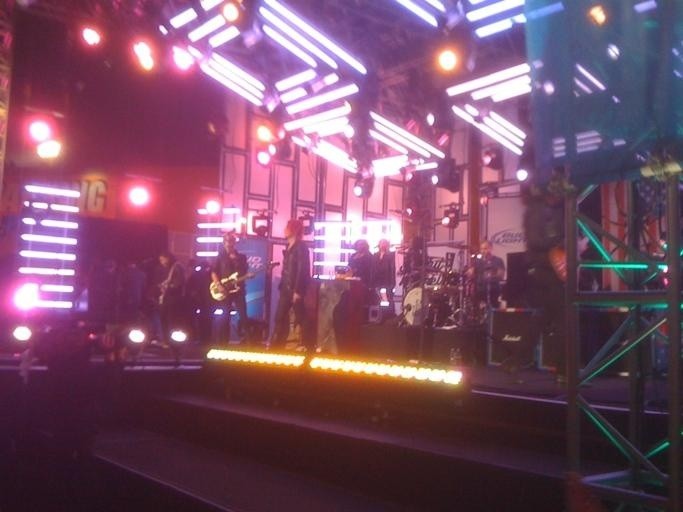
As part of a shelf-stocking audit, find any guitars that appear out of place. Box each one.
[210,262,280,301]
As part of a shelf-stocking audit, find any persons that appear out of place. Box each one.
[83,248,208,348]
[208,233,259,348]
[477,239,506,283]
[347,240,372,281]
[266,219,316,351]
[368,238,396,317]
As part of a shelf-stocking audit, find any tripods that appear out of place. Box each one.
[443,275,468,326]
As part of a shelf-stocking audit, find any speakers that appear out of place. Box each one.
[486,308,545,370]
[504,250,547,310]
[298,273,362,357]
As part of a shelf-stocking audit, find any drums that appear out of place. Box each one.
[403,287,445,325]
